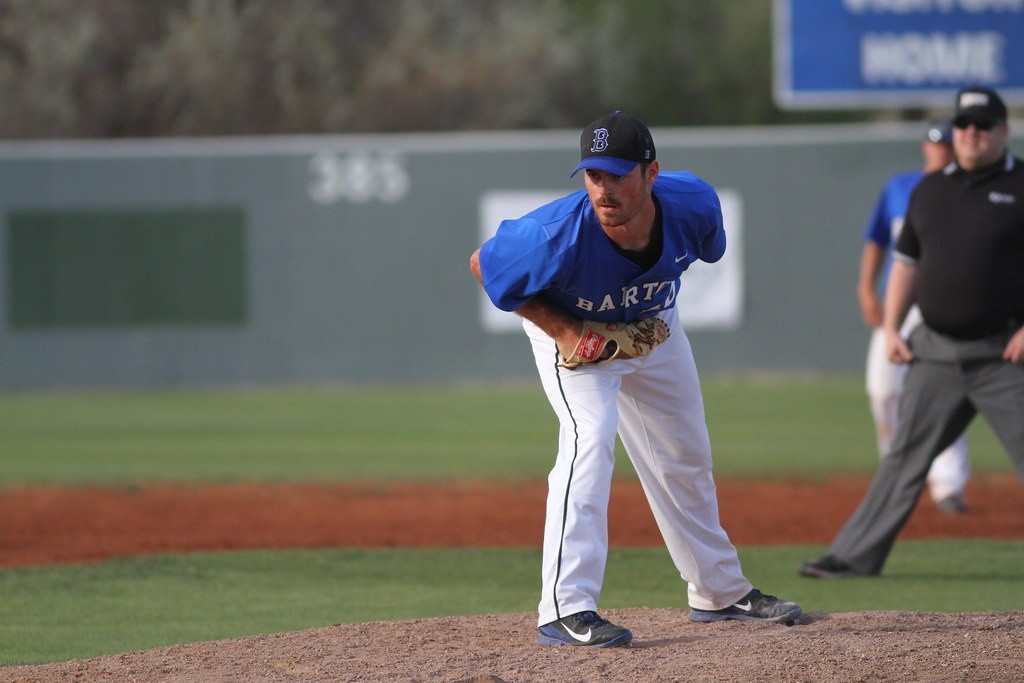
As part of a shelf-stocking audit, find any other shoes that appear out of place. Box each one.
[938,495,965,516]
[796,554,854,580]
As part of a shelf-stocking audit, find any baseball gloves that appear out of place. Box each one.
[559,317,671,369]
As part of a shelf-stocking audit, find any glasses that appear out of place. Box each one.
[953,118,995,131]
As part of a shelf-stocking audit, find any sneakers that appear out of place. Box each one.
[538,611,632,649]
[689,590,801,623]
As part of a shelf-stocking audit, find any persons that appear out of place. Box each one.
[856,122,971,513]
[472,110,803,647]
[797,86,1024,579]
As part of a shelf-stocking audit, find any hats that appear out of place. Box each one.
[925,120,951,145]
[570,110,657,178]
[950,87,1009,123]
